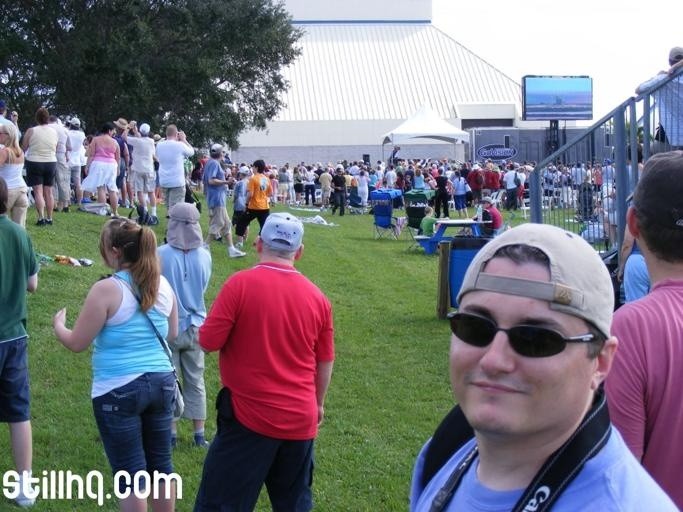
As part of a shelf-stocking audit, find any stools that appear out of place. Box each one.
[413,236,430,254]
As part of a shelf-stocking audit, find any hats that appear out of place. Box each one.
[113,118,128,129]
[140,123,151,134]
[68,118,80,128]
[669,47,683,62]
[211,144,225,152]
[167,202,204,249]
[625,151,683,229]
[239,166,250,175]
[480,197,492,204]
[456,223,615,338]
[261,212,303,252]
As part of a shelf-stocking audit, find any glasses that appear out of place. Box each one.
[447,312,597,358]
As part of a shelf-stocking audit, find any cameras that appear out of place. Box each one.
[177,132,184,135]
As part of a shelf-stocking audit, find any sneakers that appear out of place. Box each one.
[45,219,53,225]
[36,219,46,225]
[148,216,159,225]
[228,248,246,257]
[14,478,35,506]
[53,207,60,211]
[62,207,69,212]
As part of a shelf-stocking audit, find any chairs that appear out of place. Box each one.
[406,207,427,240]
[370,205,406,241]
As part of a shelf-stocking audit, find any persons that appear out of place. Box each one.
[235,159,273,249]
[230,165,251,243]
[53,211,181,512]
[82,135,94,177]
[153,133,162,204]
[451,170,468,219]
[405,158,437,206]
[79,121,121,217]
[113,117,139,209]
[156,201,213,450]
[616,194,651,305]
[203,143,247,258]
[111,135,130,207]
[634,46,682,150]
[0,122,30,230]
[0,176,43,507]
[627,143,644,206]
[503,158,615,246]
[46,114,73,213]
[122,122,159,225]
[432,168,453,219]
[183,154,209,193]
[190,211,336,512]
[21,106,59,226]
[469,157,503,206]
[603,150,683,512]
[265,145,405,221]
[419,206,450,237]
[0,99,23,139]
[155,123,195,218]
[408,222,683,512]
[471,195,502,235]
[222,151,241,190]
[437,156,468,175]
[68,117,86,207]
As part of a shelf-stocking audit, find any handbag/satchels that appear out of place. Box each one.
[173,378,184,419]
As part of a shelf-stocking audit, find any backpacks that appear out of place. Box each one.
[129,204,150,225]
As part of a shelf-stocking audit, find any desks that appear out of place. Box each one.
[430,219,493,254]
[402,189,435,206]
[370,189,402,208]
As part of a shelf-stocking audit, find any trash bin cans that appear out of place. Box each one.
[449,236,497,308]
